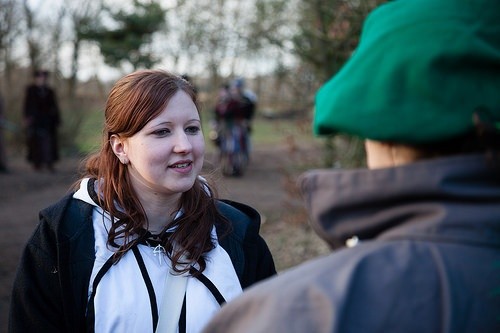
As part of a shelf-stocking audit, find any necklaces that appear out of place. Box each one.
[143,237,169,267]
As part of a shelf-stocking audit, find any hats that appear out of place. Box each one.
[312,0,500,147]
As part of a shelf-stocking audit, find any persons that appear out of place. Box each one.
[204,0,500,333]
[19,69,65,175]
[209,78,257,176]
[8,69,276,333]
